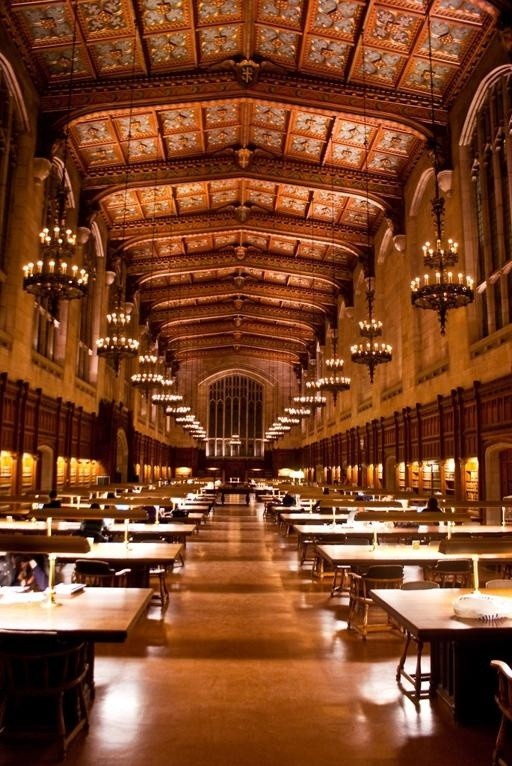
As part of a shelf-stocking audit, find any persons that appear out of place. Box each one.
[421,498,443,526]
[134,499,159,522]
[0,549,52,593]
[103,491,118,524]
[42,490,62,511]
[79,503,113,538]
[312,488,338,514]
[282,493,296,507]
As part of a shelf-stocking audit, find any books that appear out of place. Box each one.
[44,581,88,598]
